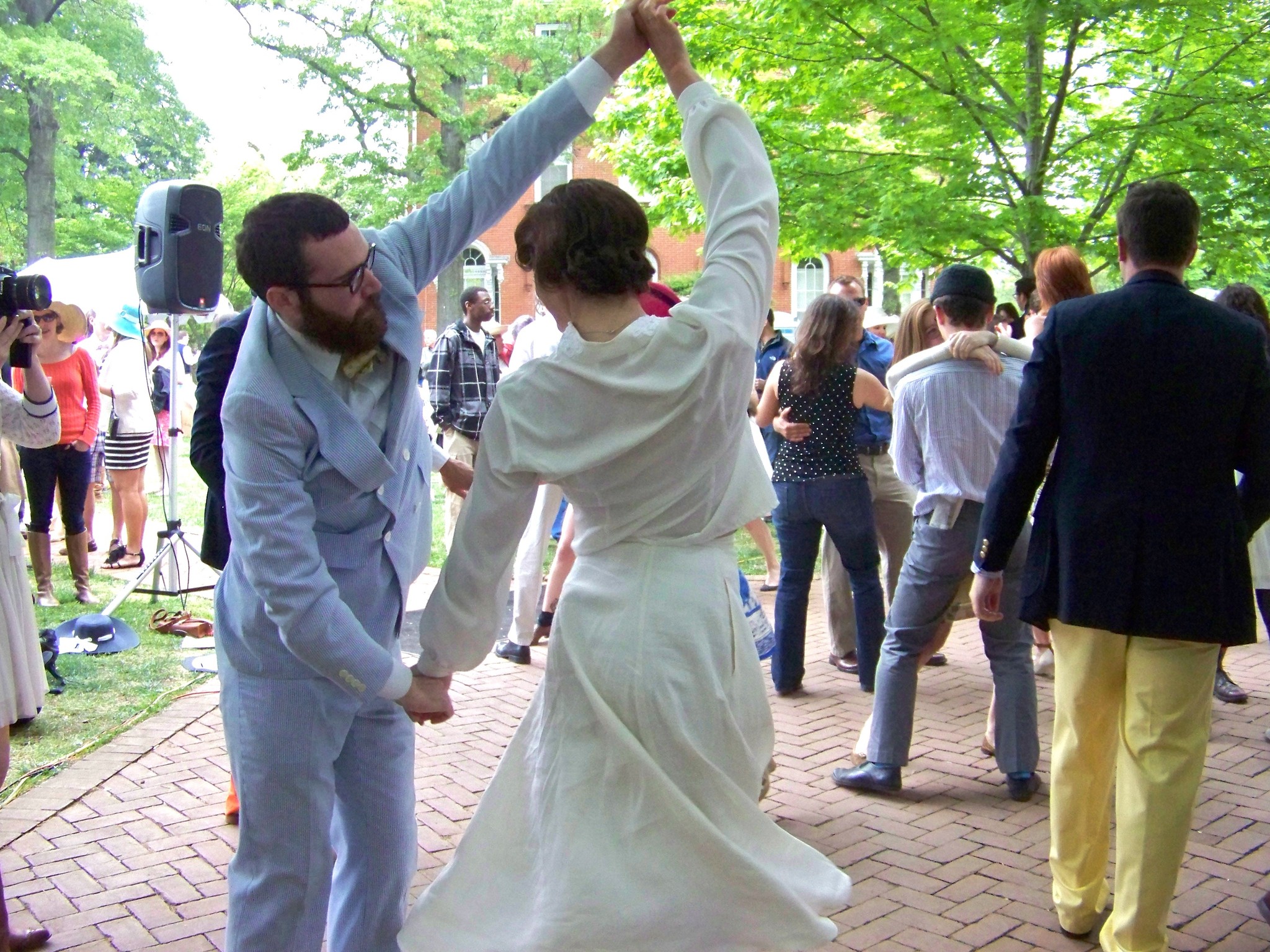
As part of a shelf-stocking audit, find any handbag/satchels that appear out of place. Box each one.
[149,609,214,638]
[107,387,119,440]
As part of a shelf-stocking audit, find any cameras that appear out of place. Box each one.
[0,266,52,322]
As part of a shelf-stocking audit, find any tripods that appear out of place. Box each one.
[98,312,227,624]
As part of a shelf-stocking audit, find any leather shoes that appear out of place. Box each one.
[1214,668,1248,702]
[1006,771,1041,802]
[925,653,948,665]
[9,927,51,952]
[108,539,123,554]
[831,762,902,795]
[59,538,97,556]
[829,649,858,674]
[495,640,532,664]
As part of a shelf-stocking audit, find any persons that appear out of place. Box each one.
[0,0,1268,952]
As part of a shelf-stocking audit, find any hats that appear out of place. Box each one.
[481,320,508,335]
[33,299,88,343]
[862,306,900,338]
[930,263,997,305]
[109,303,148,342]
[53,613,141,654]
[144,319,171,338]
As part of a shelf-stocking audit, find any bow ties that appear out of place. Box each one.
[342,340,392,379]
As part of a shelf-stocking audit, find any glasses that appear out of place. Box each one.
[853,296,866,307]
[470,300,495,306]
[34,313,57,324]
[277,243,376,294]
[150,331,166,337]
[1012,293,1019,298]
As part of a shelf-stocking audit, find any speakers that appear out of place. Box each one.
[135,179,226,315]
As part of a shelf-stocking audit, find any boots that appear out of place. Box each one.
[65,527,104,604]
[27,531,60,606]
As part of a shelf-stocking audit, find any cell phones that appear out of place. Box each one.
[8,317,32,368]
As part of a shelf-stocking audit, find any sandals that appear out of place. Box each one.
[100,545,145,569]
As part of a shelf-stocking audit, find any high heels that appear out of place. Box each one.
[529,624,551,646]
[1034,647,1054,678]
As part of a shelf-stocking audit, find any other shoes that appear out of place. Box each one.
[980,736,995,757]
[760,584,779,591]
[850,751,867,766]
